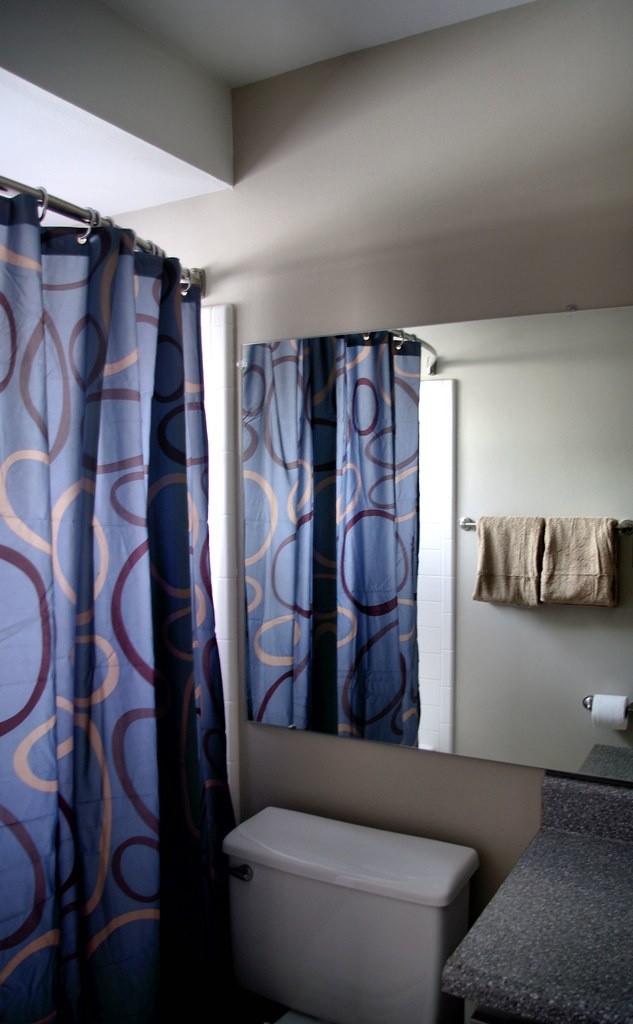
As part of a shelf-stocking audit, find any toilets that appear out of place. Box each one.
[219,805,479,1024]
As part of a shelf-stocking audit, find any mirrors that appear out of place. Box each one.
[236,308,633,786]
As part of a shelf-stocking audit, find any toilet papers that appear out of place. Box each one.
[589,694,629,732]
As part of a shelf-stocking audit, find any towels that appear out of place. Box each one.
[472,516,544,610]
[539,518,619,606]
